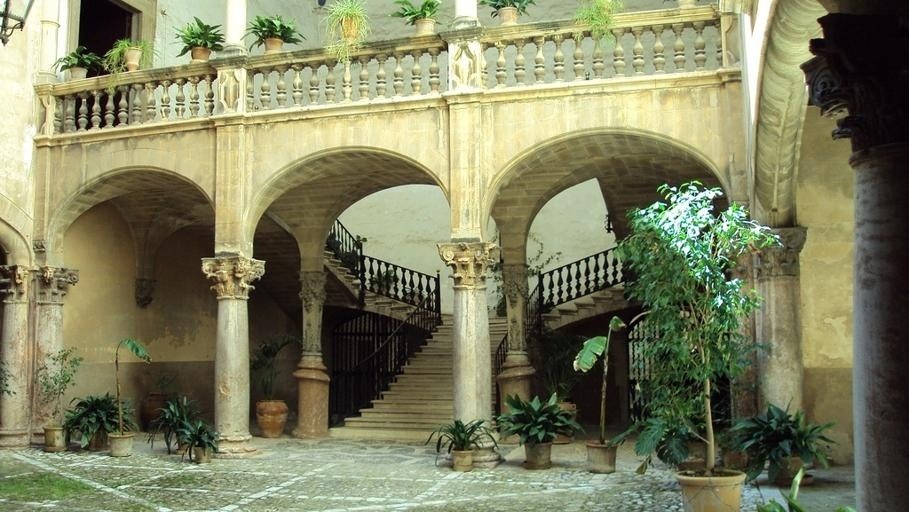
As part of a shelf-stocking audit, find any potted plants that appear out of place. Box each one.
[178,419,223,464]
[174,16,226,61]
[390,0,442,36]
[34,347,83,446]
[52,46,101,79]
[250,335,302,438]
[493,391,586,469]
[425,416,497,472]
[100,39,159,97]
[480,0,537,26]
[146,391,201,455]
[318,0,373,63]
[572,315,620,474]
[614,178,783,512]
[61,391,139,451]
[106,333,151,456]
[239,13,308,53]
[662,0,700,7]
[726,397,842,492]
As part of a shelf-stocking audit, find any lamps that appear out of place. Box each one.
[0,0,35,47]
